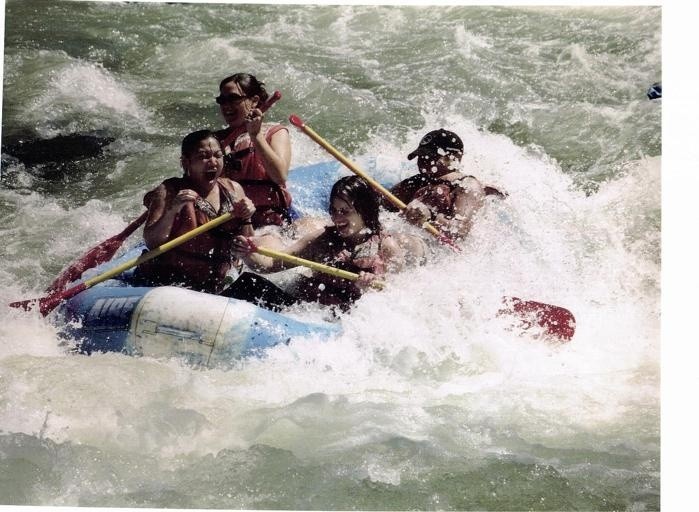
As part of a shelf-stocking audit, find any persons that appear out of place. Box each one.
[218,172,405,316]
[380,129,485,240]
[130,123,257,289]
[142,72,293,226]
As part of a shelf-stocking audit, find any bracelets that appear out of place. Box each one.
[241,221,252,226]
[428,208,439,224]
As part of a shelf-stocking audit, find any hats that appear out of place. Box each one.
[408,129,464,160]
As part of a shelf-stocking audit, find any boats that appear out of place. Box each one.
[54,156,487,382]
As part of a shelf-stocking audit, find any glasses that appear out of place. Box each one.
[328,204,352,217]
[216,92,251,105]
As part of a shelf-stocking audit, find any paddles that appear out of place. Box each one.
[43,92,281,296]
[8,202,241,316]
[242,239,576,344]
